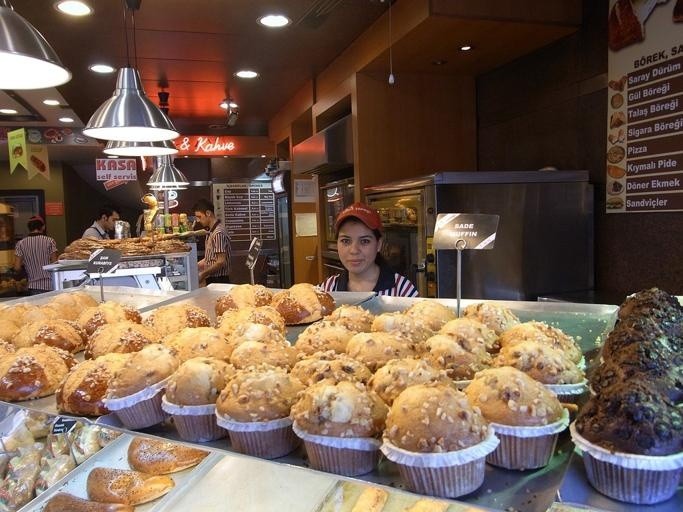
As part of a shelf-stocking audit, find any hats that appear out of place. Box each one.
[28,215,45,225]
[335,203,384,236]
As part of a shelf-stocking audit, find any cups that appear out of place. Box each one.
[160,393,229,444]
[28,131,39,139]
[102,374,176,429]
[154,212,187,236]
[291,410,382,479]
[214,406,303,460]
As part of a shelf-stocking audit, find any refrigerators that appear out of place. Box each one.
[271,170,320,288]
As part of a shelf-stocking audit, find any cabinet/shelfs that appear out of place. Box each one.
[42,241,200,293]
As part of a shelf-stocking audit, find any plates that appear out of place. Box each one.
[27,138,40,143]
[57,259,88,266]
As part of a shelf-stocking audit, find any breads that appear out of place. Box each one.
[12,144,22,156]
[59,235,192,259]
[0,283,683,511]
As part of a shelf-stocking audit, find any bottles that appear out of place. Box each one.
[567,420,683,504]
[383,429,501,498]
[485,418,572,472]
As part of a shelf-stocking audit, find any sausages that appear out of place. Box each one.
[31,155,46,172]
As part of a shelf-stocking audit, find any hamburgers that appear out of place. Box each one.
[606,197,622,208]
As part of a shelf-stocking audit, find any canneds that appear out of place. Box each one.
[155,214,188,235]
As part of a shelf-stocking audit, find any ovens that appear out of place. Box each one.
[319,176,355,278]
[363,165,595,304]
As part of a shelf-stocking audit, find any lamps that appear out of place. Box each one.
[222,96,239,128]
[251,159,275,181]
[81,0,190,192]
[0,0,73,92]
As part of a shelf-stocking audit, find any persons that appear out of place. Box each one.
[195,200,234,288]
[14,215,59,295]
[311,202,419,298]
[82,208,120,242]
